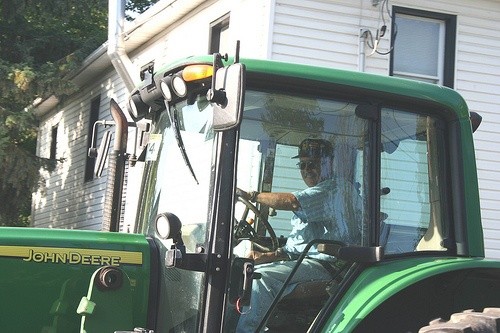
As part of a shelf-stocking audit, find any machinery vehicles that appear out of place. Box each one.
[0,40,500,333]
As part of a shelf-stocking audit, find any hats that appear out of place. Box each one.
[290,139,334,159]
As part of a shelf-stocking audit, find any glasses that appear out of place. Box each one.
[296,160,322,170]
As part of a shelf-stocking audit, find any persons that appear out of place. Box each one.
[236,139,365,333]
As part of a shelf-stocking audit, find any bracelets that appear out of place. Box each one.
[250,191,259,202]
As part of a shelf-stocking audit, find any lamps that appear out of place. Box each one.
[128,64,215,119]
